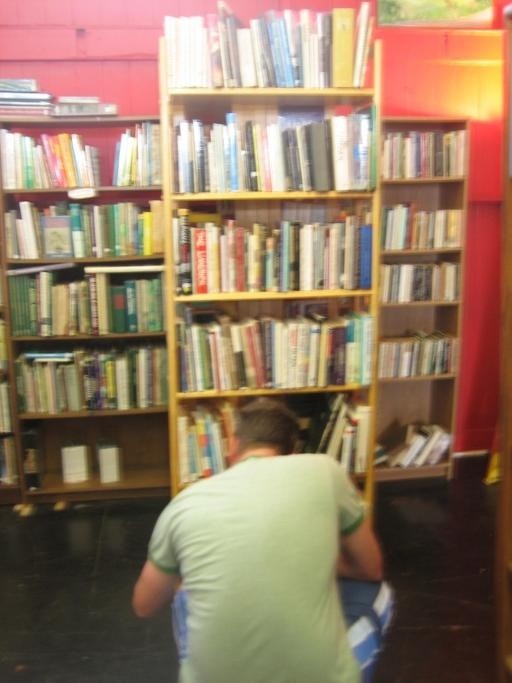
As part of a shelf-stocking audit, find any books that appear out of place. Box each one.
[2,125,162,190]
[4,264,157,336]
[0,78,118,117]
[375,422,451,469]
[178,395,238,482]
[381,130,462,183]
[300,395,369,476]
[16,347,169,414]
[172,104,376,193]
[179,308,372,392]
[0,314,20,490]
[375,327,447,377]
[380,261,458,303]
[4,201,162,260]
[379,200,462,252]
[173,209,368,298]
[162,2,369,92]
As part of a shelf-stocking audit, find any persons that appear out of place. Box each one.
[131,399,395,683]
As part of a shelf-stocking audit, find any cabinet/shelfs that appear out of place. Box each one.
[1,286,24,498]
[0,114,170,508]
[375,114,469,483]
[157,35,381,518]
